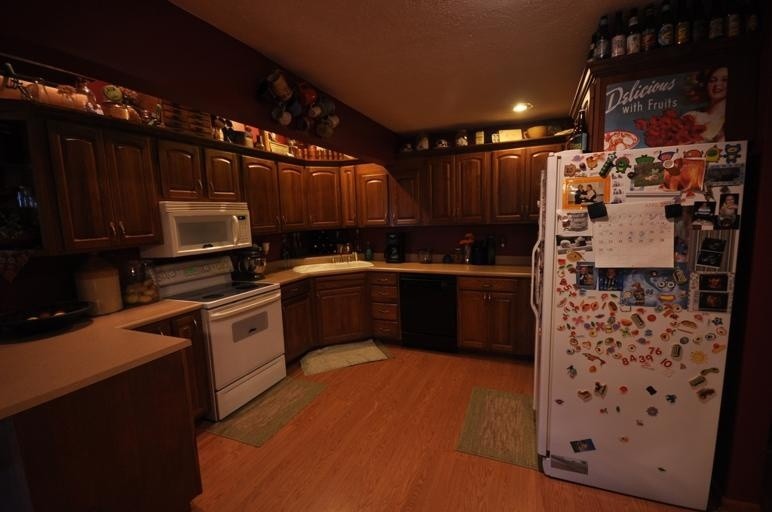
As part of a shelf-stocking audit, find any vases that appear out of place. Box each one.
[369,271,399,341]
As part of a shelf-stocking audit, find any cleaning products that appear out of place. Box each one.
[364,241,375,261]
[443,251,453,264]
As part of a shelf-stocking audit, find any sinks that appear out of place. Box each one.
[293,260,374,274]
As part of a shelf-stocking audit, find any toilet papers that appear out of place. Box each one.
[31,83,89,111]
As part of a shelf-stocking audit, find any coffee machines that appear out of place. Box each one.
[462,234,496,264]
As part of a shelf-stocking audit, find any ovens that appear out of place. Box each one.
[202,286,287,422]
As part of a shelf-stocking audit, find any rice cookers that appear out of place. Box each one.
[76,264,125,316]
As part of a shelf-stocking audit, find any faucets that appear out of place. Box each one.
[332,252,358,263]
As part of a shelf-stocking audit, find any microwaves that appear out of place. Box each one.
[140,200,254,259]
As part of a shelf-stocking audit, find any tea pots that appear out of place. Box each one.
[238,243,266,279]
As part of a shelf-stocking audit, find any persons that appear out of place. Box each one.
[573,183,586,203]
[679,68,728,142]
[695,203,711,223]
[582,184,597,204]
[719,195,739,227]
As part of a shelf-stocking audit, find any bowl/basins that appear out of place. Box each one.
[3,300,93,335]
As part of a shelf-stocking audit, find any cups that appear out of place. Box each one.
[523,125,552,140]
[423,255,432,264]
[269,73,340,137]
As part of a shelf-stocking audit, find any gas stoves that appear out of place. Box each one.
[165,281,281,304]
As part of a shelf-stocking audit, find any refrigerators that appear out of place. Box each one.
[527,141,747,512]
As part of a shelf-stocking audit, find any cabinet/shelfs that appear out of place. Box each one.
[341,158,356,227]
[131,309,211,427]
[313,272,371,346]
[243,148,304,232]
[489,136,569,226]
[304,160,342,229]
[570,50,733,152]
[156,133,243,203]
[456,273,536,358]
[282,279,313,364]
[355,151,423,229]
[424,143,490,225]
[1,103,161,250]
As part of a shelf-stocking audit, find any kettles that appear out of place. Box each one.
[384,243,406,263]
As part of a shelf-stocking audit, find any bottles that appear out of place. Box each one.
[255,135,266,151]
[365,244,373,260]
[76,78,98,111]
[288,140,343,161]
[593,1,759,62]
[344,243,352,254]
[455,248,462,263]
[443,255,451,263]
[281,239,289,260]
[569,110,588,153]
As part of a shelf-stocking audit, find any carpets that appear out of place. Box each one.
[300,341,394,377]
[207,374,328,449]
[456,384,540,472]
